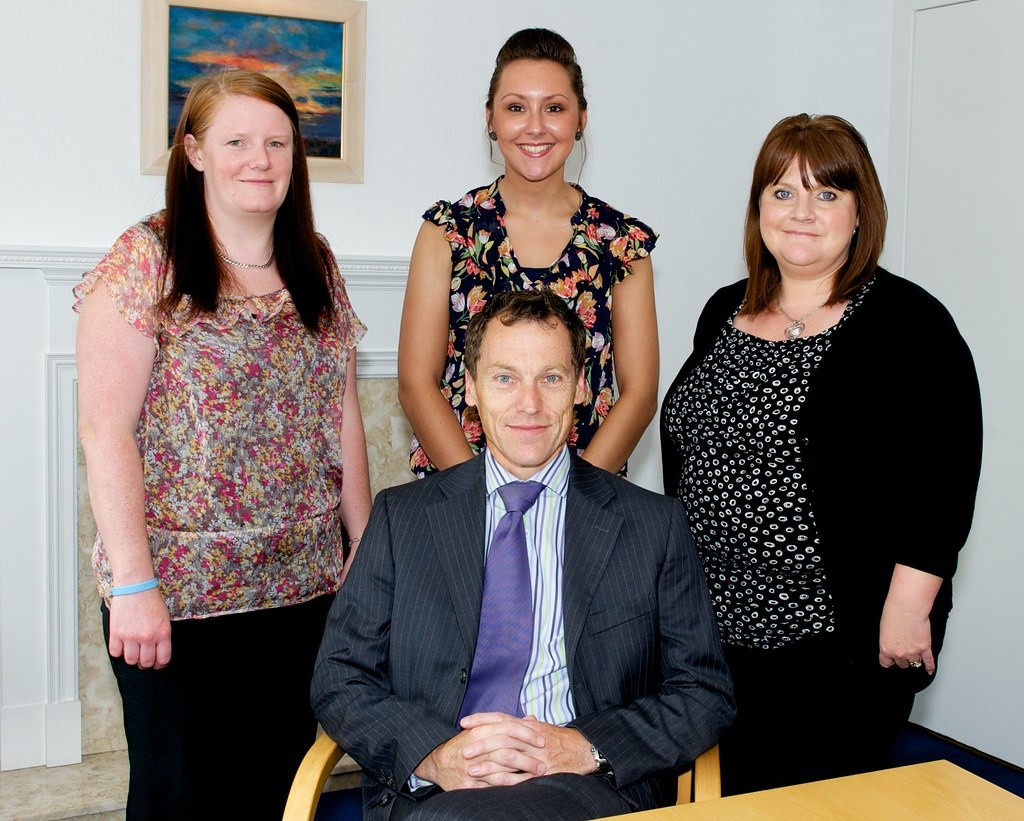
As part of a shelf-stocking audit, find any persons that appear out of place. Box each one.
[310,289,738,821]
[397,28,660,483]
[72,69,373,821]
[659,113,984,798]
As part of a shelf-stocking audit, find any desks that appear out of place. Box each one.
[585,759,1024,821]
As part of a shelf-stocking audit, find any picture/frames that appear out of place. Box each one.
[141,0,367,184]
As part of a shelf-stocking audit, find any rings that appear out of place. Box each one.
[910,656,922,668]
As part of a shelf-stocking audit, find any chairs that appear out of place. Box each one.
[281,730,721,821]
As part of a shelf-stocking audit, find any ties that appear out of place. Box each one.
[455,481,545,731]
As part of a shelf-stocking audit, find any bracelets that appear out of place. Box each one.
[109,576,159,596]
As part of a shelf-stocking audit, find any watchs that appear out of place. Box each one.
[590,744,611,772]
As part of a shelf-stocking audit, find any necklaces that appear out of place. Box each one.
[215,245,275,271]
[775,296,826,341]
